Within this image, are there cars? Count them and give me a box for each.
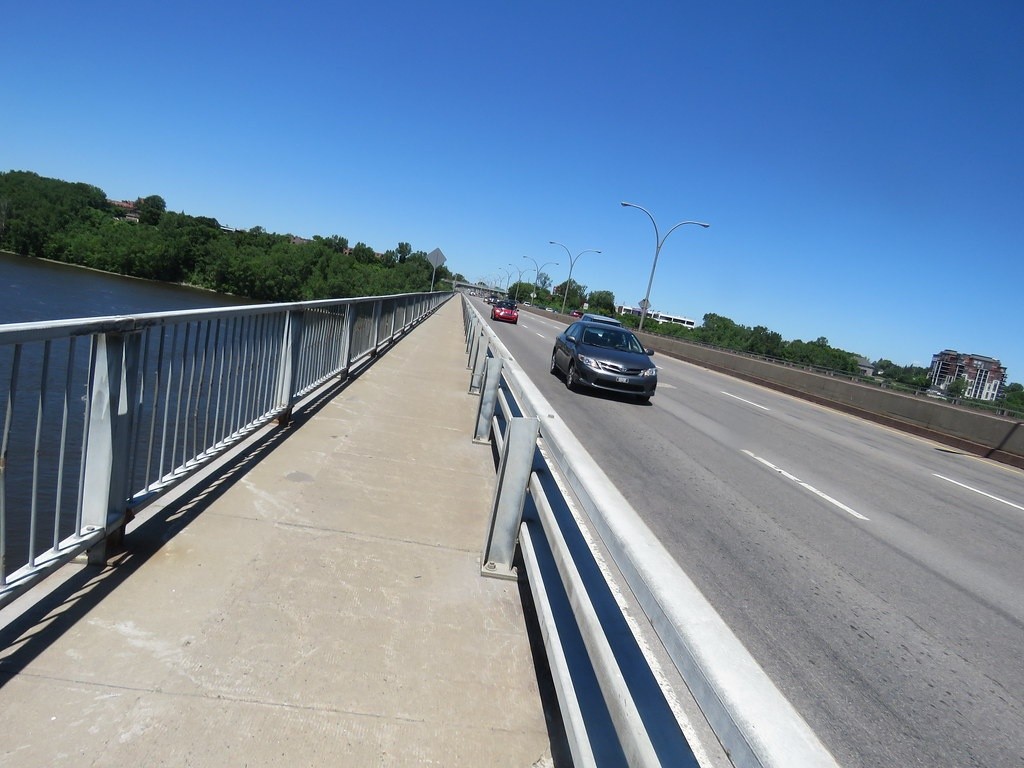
[467,290,518,309]
[491,301,520,324]
[546,307,560,314]
[523,301,531,307]
[569,310,583,318]
[549,320,658,403]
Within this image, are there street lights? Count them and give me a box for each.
[549,241,602,314]
[620,201,710,332]
[473,267,522,301]
[522,255,560,306]
[509,263,537,301]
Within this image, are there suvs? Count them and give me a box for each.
[568,313,626,344]
